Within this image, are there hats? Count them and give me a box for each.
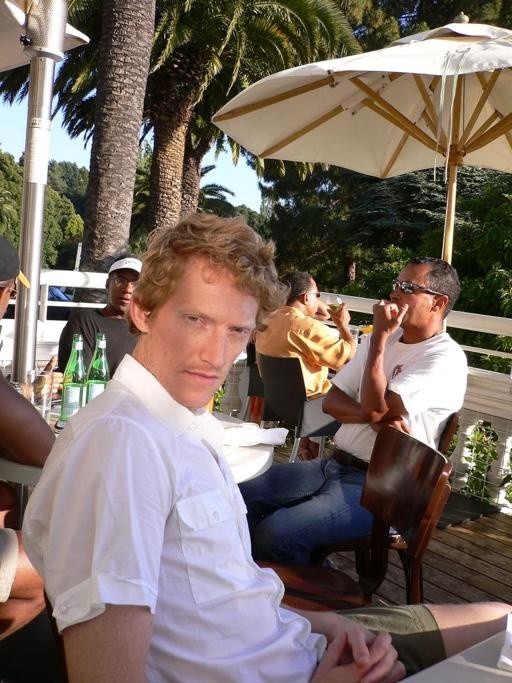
[0,236,30,288]
[109,258,142,274]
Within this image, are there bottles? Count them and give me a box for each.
[61,333,87,422]
[87,332,110,404]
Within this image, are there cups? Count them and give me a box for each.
[26,369,53,425]
[327,294,344,314]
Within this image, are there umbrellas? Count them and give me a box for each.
[210,11,512,266]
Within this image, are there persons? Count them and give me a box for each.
[60,255,143,380]
[21,210,512,682]
[1,235,55,645]
[234,257,469,611]
[255,268,357,401]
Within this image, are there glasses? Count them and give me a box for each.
[391,279,444,296]
[110,277,138,287]
[2,287,18,299]
[297,292,320,298]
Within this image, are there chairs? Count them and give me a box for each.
[256,424,453,611]
[317,411,460,596]
[241,345,265,420]
[256,353,340,461]
